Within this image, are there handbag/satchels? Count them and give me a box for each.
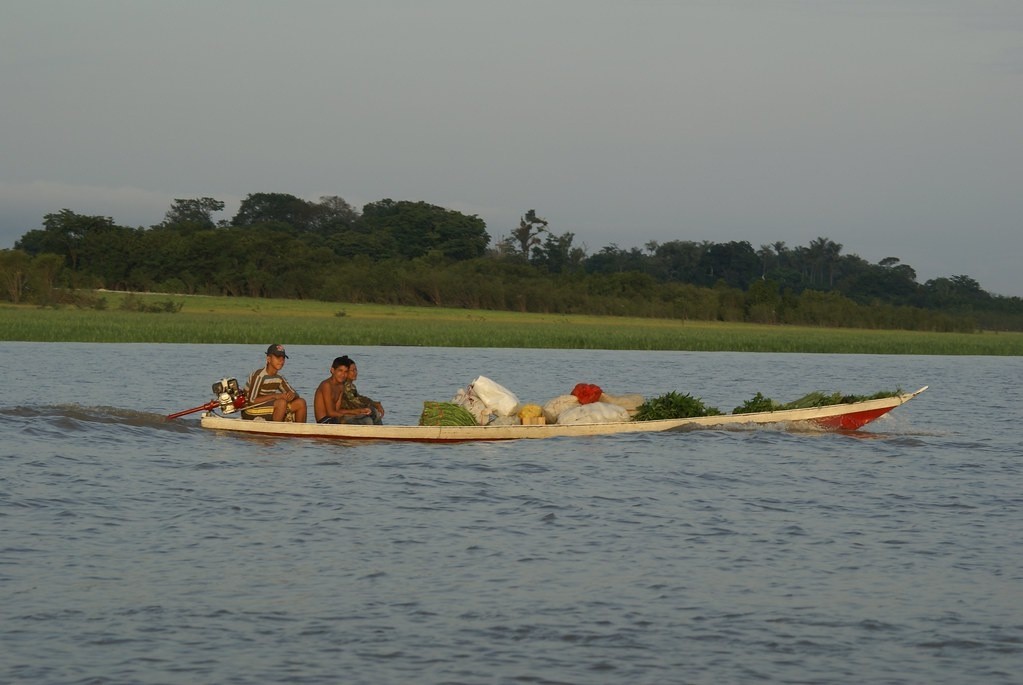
[472,375,520,417]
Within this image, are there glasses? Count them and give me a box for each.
[349,368,357,373]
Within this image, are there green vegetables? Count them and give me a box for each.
[421,400,476,426]
[631,391,727,421]
[732,386,903,414]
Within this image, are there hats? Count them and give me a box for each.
[265,344,289,359]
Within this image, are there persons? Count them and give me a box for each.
[241,344,307,422]
[343,358,385,425]
[314,357,374,424]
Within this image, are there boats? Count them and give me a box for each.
[162,377,933,444]
